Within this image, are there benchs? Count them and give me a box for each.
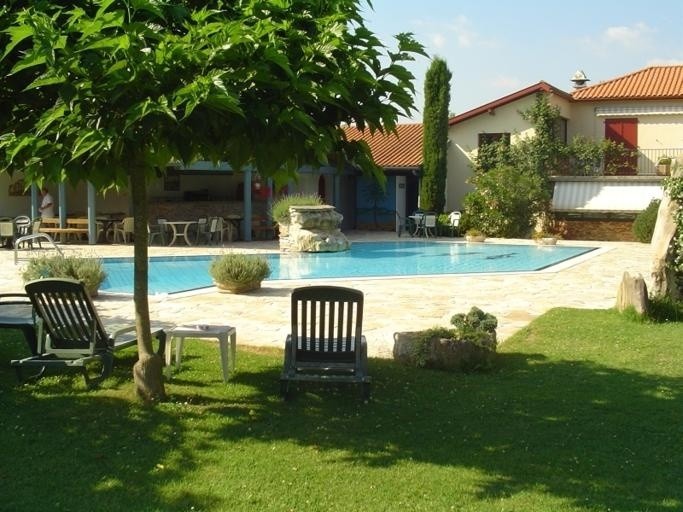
[253,217,276,239]
[38,217,90,245]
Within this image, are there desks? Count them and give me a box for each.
[172,322,237,382]
[163,220,197,246]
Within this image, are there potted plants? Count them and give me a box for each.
[210,250,271,294]
[541,232,560,245]
[656,153,673,176]
[465,228,486,242]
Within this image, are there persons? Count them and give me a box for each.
[38,186,55,237]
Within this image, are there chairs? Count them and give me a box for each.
[279,285,372,404]
[198,214,242,244]
[395,210,462,241]
[1,216,30,249]
[10,277,166,390]
[148,215,170,246]
[94,215,135,243]
